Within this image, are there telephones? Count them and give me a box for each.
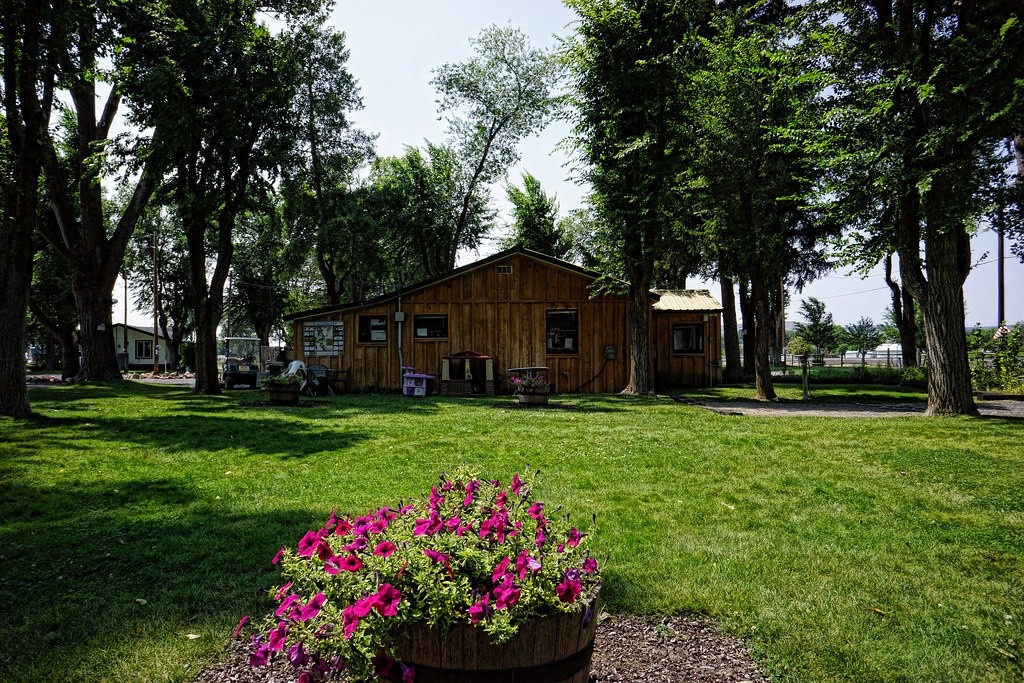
[605,345,616,360]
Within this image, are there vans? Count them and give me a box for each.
[28,347,60,367]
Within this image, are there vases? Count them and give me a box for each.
[518,387,550,407]
[390,576,599,683]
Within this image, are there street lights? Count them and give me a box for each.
[135,231,158,374]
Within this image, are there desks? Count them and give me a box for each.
[303,368,351,394]
[507,366,550,395]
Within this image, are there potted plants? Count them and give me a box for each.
[261,375,303,406]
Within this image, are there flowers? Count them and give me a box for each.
[232,472,610,683]
[507,371,551,389]
